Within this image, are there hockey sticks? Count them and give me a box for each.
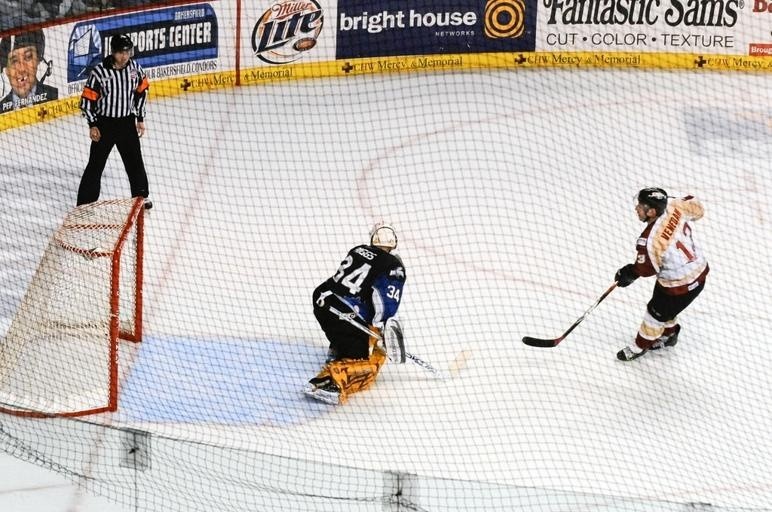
[523,195,694,347]
[331,307,474,383]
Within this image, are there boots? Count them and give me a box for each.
[617,324,681,362]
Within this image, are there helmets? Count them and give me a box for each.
[0,28,45,73]
[111,33,134,52]
[637,186,668,217]
[371,226,397,249]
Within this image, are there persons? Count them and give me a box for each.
[0,0,156,27]
[74,34,153,210]
[0,29,58,114]
[303,226,406,404]
[614,187,711,362]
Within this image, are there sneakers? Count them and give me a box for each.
[303,376,343,404]
[615,263,640,288]
[143,198,153,210]
